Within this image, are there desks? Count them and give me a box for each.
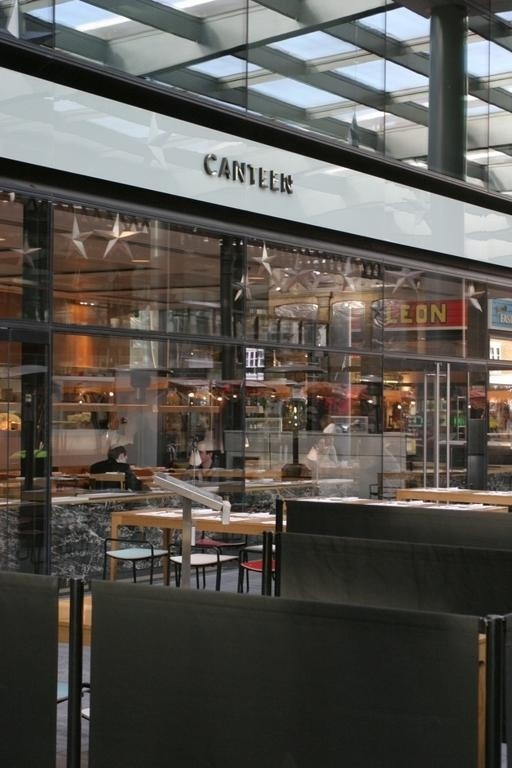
[110,507,287,586]
[288,486,512,514]
[377,467,512,500]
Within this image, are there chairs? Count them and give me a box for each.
[56,474,126,491]
[369,484,396,501]
[103,531,277,594]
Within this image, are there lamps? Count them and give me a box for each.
[306,438,319,483]
[189,438,201,486]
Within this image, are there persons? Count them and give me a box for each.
[181,440,227,482]
[308,437,342,466]
[90,444,142,491]
